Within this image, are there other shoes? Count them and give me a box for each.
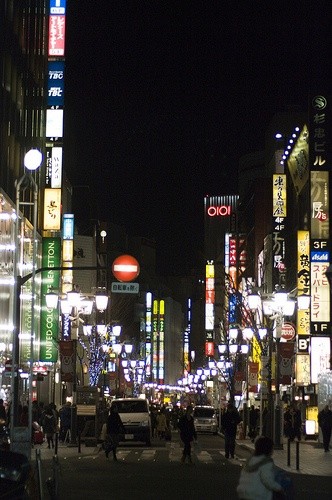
[187,458,192,464]
[181,455,185,462]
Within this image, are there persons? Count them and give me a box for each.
[149,402,186,442]
[317,405,332,453]
[215,405,301,443]
[0,397,77,449]
[220,398,242,458]
[104,404,127,461]
[178,405,198,465]
[237,434,283,500]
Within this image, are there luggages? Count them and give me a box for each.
[34,427,43,444]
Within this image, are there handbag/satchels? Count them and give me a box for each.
[193,440,201,454]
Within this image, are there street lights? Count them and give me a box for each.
[10,149,43,468]
[44,282,110,448]
[178,287,311,449]
[102,325,147,398]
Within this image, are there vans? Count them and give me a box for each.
[191,406,218,436]
[109,398,152,446]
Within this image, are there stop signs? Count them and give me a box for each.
[112,255,140,283]
